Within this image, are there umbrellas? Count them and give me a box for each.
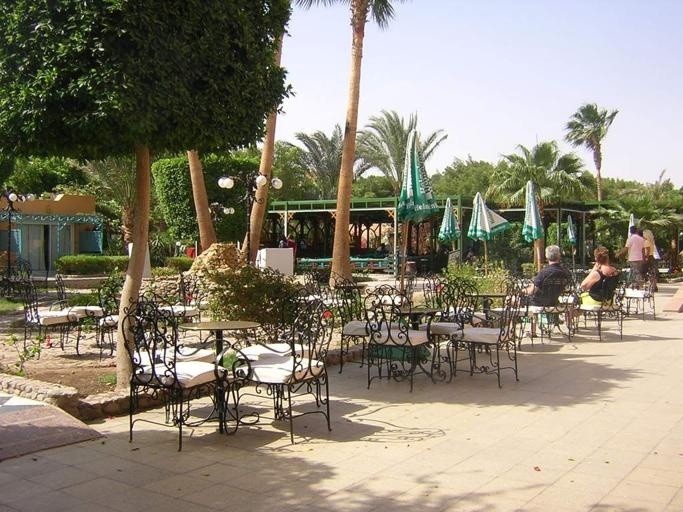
[467,191,513,276]
[566,214,577,265]
[521,179,545,274]
[396,129,440,306]
[437,196,463,251]
[626,212,636,242]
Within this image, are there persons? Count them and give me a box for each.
[613,226,644,291]
[277,234,286,248]
[286,234,297,272]
[565,245,617,329]
[349,228,367,249]
[504,244,572,338]
[636,229,660,293]
